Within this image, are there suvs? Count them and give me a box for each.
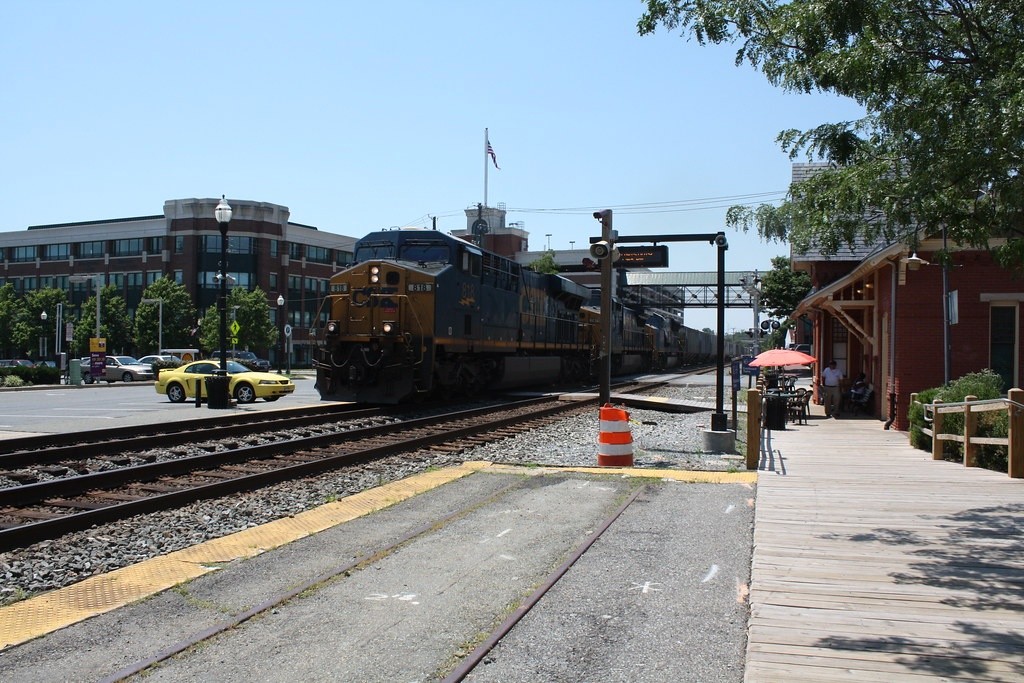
[211,351,270,372]
[0,360,34,368]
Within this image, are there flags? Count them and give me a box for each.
[487,140,499,169]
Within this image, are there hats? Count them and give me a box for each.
[827,361,837,365]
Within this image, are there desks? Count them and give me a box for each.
[762,394,803,424]
[766,390,797,419]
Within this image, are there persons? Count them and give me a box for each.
[820,360,844,418]
[841,372,865,411]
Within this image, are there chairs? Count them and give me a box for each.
[765,375,814,425]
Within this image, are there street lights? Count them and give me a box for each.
[69,274,106,384]
[215,194,233,376]
[276,295,285,374]
[143,299,162,354]
[900,215,950,390]
[39,311,47,360]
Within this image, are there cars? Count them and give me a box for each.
[80,355,150,382]
[138,355,183,369]
[156,359,293,402]
[35,360,57,368]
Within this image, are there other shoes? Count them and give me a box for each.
[836,416,840,419]
[825,415,830,419]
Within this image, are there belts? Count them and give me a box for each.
[824,385,837,387]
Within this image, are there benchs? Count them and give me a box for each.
[850,388,874,417]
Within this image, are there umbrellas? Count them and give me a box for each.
[749,347,817,391]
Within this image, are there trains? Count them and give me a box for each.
[314,230,751,408]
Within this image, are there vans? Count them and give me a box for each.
[158,348,204,365]
